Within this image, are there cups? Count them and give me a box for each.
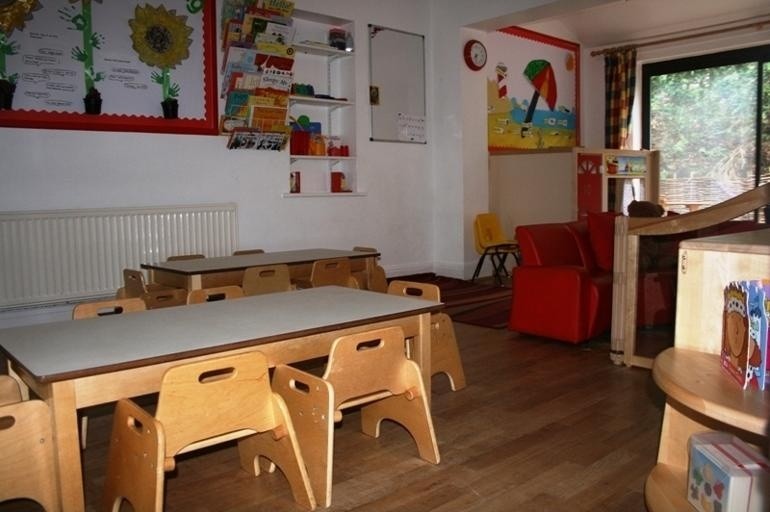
[290,172,300,193]
[331,171,345,192]
[341,145,349,157]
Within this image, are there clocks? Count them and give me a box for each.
[464,39,488,71]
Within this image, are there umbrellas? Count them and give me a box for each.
[521,60,556,138]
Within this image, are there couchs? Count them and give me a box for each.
[509,218,763,347]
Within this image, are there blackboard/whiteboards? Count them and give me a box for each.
[368,24,427,145]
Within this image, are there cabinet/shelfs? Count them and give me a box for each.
[573,147,662,217]
[220,0,365,197]
[644,348,770,512]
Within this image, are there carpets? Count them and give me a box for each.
[387,273,512,329]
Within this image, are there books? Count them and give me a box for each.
[218,0,296,151]
[687,431,770,512]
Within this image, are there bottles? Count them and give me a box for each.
[345,32,354,52]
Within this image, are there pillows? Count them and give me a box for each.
[587,210,623,274]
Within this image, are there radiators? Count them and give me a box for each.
[0,203,240,311]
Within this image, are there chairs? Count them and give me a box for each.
[471,213,519,287]
